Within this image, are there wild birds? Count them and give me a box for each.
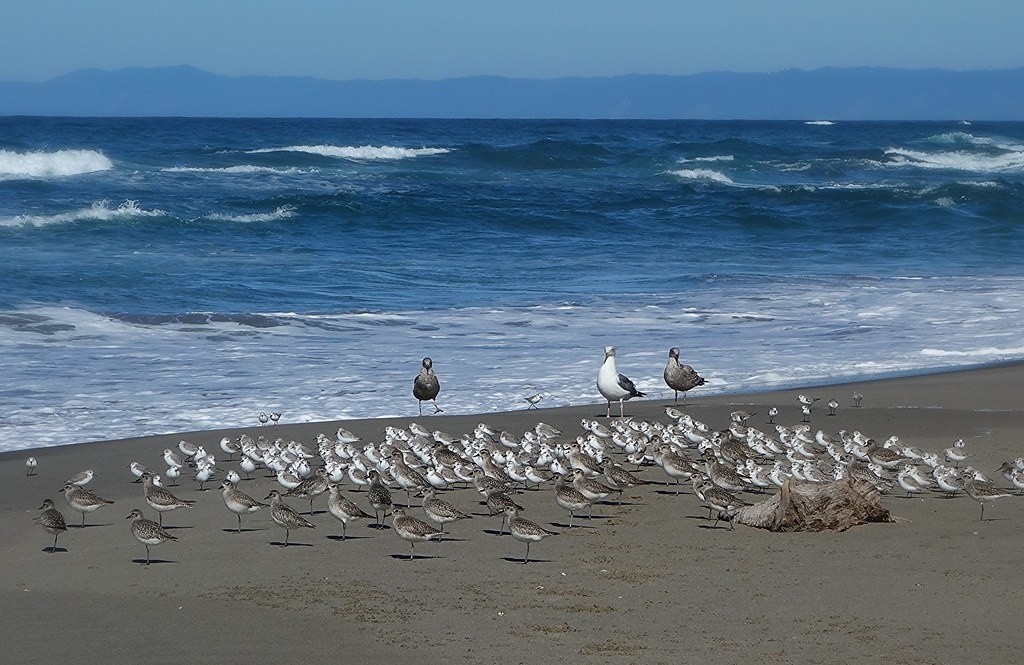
[796,389,863,421]
[324,483,377,541]
[263,490,317,547]
[490,505,560,563]
[26,456,38,475]
[259,411,281,427]
[664,347,708,408]
[652,403,1024,534]
[333,417,651,529]
[412,357,444,416]
[413,486,474,542]
[59,483,114,527]
[384,506,450,562]
[524,394,544,410]
[33,499,68,553]
[125,509,178,564]
[597,345,647,419]
[126,434,334,533]
